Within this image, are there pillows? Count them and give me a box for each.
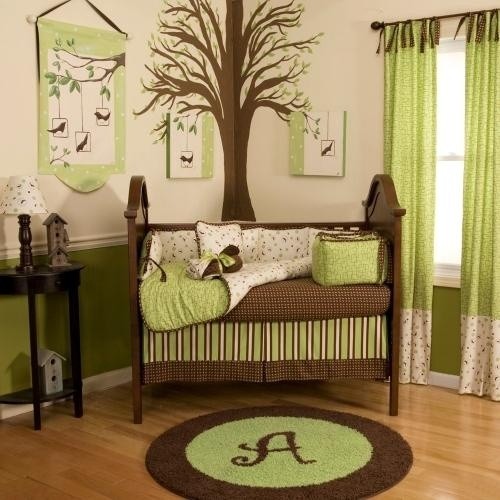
[137,228,162,281]
[195,220,245,259]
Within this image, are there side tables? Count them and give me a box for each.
[0,261,87,431]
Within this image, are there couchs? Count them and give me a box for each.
[124,174,406,425]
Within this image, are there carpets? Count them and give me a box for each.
[145,405,413,500]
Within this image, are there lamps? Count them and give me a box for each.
[0,175,48,275]
